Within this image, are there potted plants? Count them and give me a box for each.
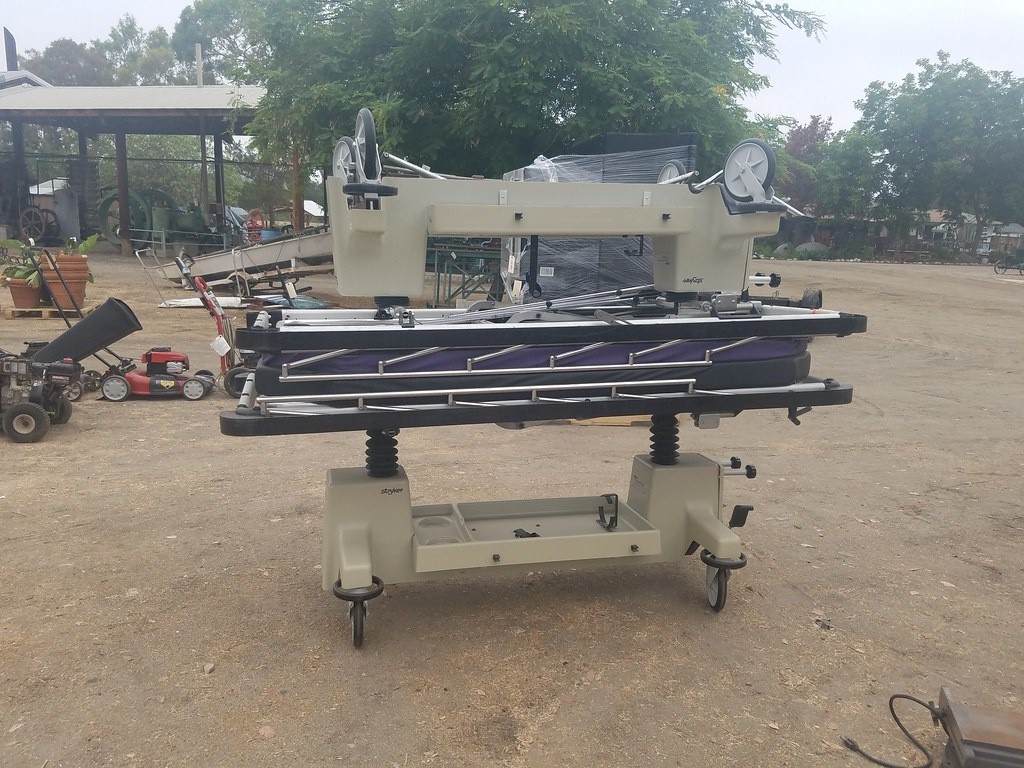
[0,231,100,308]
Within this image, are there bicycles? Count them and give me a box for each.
[994,249,1024,275]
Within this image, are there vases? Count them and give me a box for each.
[41,253,90,309]
